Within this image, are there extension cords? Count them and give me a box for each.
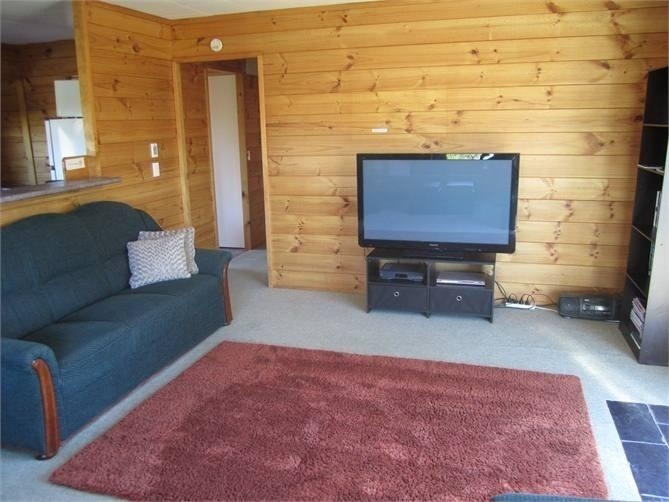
[505,299,535,309]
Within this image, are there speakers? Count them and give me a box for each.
[558,295,618,321]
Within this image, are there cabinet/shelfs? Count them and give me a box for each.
[366,252,493,322]
[618,66,669,366]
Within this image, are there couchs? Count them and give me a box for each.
[1,201,232,460]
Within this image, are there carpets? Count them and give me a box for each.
[609,401,668,501]
[51,339,609,502]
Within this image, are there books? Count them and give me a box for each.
[637,163,664,171]
[629,295,646,352]
[647,242,654,298]
[651,189,663,242]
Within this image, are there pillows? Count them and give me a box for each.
[138,226,198,275]
[127,234,191,289]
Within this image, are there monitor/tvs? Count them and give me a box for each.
[357,153,520,259]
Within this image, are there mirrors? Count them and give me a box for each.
[1,0,89,196]
